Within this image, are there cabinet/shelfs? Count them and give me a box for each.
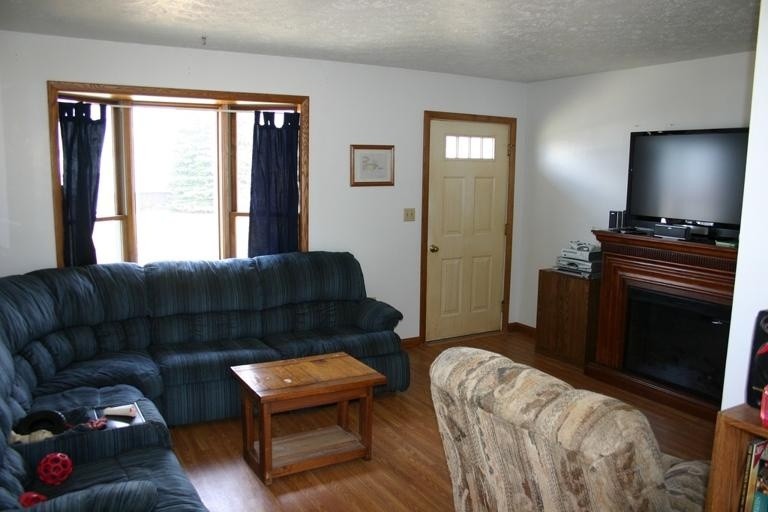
[535,265,597,366]
[587,228,738,421]
[704,401,768,511]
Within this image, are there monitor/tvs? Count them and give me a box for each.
[625,127,750,245]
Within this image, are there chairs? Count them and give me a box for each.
[427,345,713,511]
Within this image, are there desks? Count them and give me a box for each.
[224,347,389,484]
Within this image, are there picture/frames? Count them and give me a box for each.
[349,143,396,189]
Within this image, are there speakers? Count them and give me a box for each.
[608,211,622,231]
[623,210,635,231]
[653,224,691,241]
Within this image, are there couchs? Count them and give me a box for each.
[3,249,413,511]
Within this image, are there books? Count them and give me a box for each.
[737,440,768,512]
